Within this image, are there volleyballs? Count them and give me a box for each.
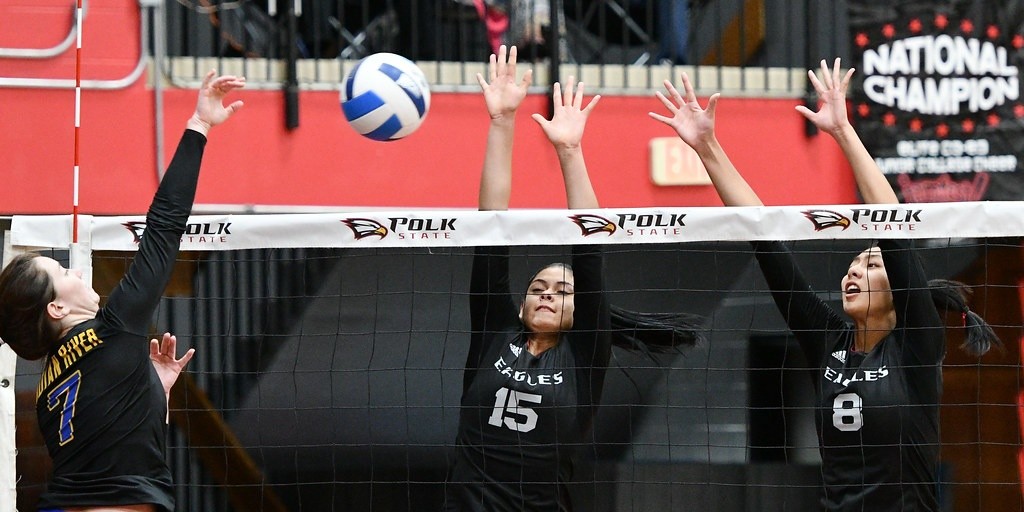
[339,53,431,143]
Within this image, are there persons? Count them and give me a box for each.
[0,69,245,512]
[648,58,1003,512]
[387,0,696,67]
[444,44,706,511]
